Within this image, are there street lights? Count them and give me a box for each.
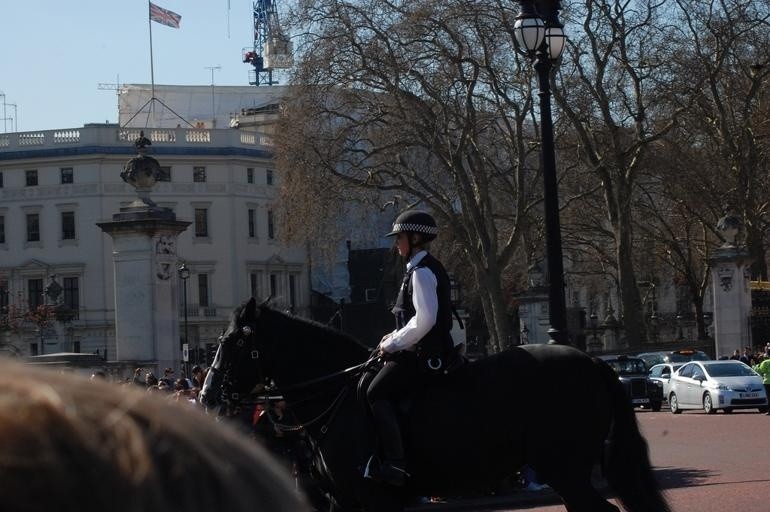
[522,321,529,345]
[590,312,599,343]
[514,0,568,346]
[180,261,191,345]
[642,280,713,342]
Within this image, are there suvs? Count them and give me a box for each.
[599,353,663,412]
[636,347,712,372]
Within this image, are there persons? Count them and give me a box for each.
[85,364,205,401]
[356,210,455,480]
[720,343,770,413]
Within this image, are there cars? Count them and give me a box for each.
[648,362,689,401]
[666,360,769,415]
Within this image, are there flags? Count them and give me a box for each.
[151,3,182,28]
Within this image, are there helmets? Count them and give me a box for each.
[385,210,439,239]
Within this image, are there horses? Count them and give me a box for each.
[197,293,676,512]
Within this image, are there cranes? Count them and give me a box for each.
[242,0,298,87]
